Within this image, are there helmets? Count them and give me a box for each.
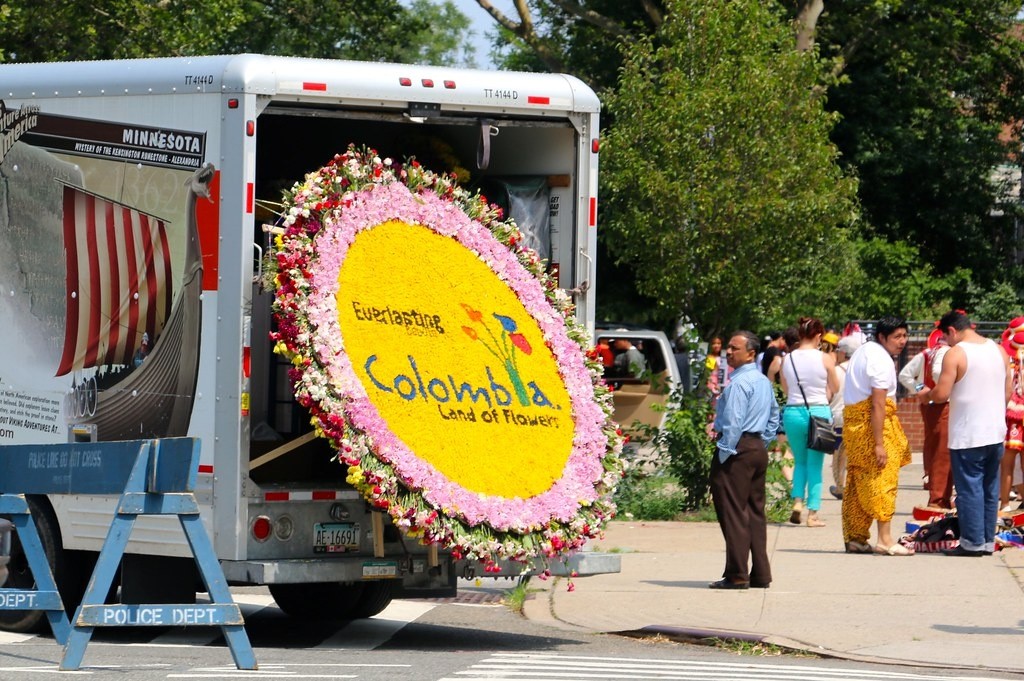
[822,332,839,346]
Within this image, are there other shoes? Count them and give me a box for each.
[943,546,982,557]
[807,518,826,527]
[830,485,843,499]
[708,578,750,589]
[789,503,802,525]
[983,550,993,556]
[750,582,769,588]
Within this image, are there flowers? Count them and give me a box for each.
[258,144,639,600]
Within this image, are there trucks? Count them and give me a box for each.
[0,51,602,645]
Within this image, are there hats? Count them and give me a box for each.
[1004,314,1024,337]
[1003,331,1024,359]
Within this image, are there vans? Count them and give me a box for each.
[592,326,686,476]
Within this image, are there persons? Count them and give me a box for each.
[702,317,915,589]
[1002,316,1024,526]
[898,309,1012,558]
[443,153,510,221]
[598,326,688,380]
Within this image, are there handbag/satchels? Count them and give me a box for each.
[806,414,836,455]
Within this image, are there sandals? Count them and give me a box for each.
[846,541,875,554]
[875,542,915,556]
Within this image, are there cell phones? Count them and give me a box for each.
[916,384,933,404]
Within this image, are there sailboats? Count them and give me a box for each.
[52,160,215,442]
